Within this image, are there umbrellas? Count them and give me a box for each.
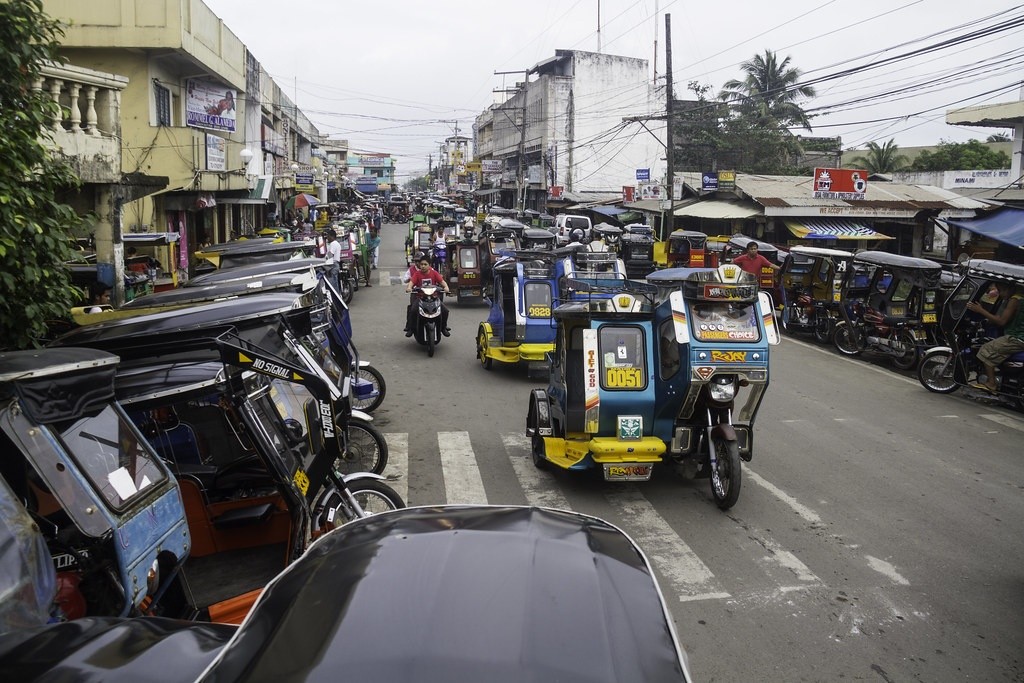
[285,193,321,209]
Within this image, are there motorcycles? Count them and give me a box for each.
[404,283,460,355]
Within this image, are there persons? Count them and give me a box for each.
[303,218,313,232]
[586,231,608,252]
[199,235,211,250]
[325,229,341,269]
[228,230,237,243]
[298,211,303,221]
[126,247,136,259]
[966,281,1024,389]
[731,242,780,284]
[309,207,327,221]
[392,205,398,224]
[403,252,451,337]
[464,212,477,235]
[89,282,111,313]
[566,234,582,247]
[431,225,448,249]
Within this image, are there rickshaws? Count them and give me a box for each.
[915,259,1024,410]
[779,244,856,346]
[520,263,793,508]
[831,249,945,373]
[48,182,786,574]
[0,348,210,659]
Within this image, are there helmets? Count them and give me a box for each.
[411,252,425,261]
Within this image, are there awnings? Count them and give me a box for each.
[783,217,896,239]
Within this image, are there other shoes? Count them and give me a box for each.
[404,327,409,331]
[441,329,450,337]
[445,326,451,331]
[405,329,413,337]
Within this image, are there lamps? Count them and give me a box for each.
[239,149,254,170]
[290,163,299,174]
